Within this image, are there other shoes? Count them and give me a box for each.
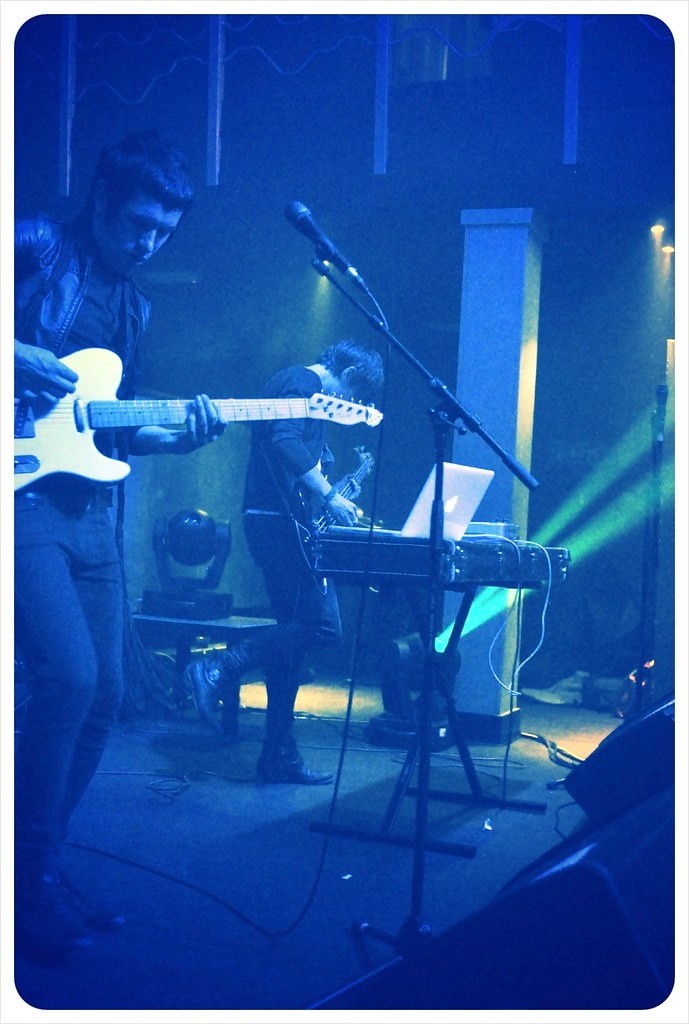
[13,876,79,962]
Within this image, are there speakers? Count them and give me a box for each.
[302,784,674,1009]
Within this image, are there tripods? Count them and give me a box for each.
[308,331,547,944]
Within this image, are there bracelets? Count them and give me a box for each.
[320,487,337,510]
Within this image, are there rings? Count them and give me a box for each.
[217,418,228,430]
[352,518,358,523]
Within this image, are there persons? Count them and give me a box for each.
[13,130,226,951]
[182,338,387,786]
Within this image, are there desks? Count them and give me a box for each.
[131,614,279,741]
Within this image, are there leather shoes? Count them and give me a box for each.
[182,653,224,735]
[257,752,333,784]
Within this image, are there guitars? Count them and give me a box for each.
[14,347,385,493]
[288,446,376,602]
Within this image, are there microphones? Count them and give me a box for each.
[310,257,371,326]
[285,199,369,296]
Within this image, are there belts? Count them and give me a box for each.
[24,482,114,517]
